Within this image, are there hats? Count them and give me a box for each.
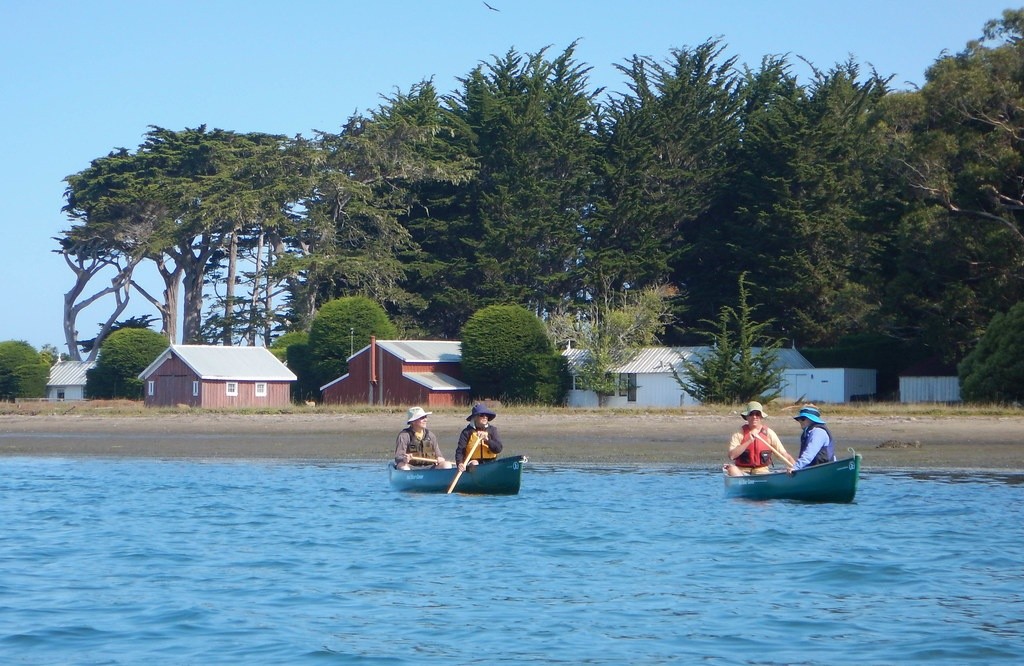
[741,401,769,421]
[466,405,496,421]
[793,407,826,426]
[407,407,433,425]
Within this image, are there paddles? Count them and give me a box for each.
[446,435,484,495]
[751,432,794,468]
[405,453,457,465]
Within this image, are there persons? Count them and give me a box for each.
[727,401,795,476]
[787,406,836,476]
[395,407,452,470]
[455,404,502,472]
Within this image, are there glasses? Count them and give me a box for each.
[749,413,760,416]
[796,418,806,422]
[478,414,488,417]
